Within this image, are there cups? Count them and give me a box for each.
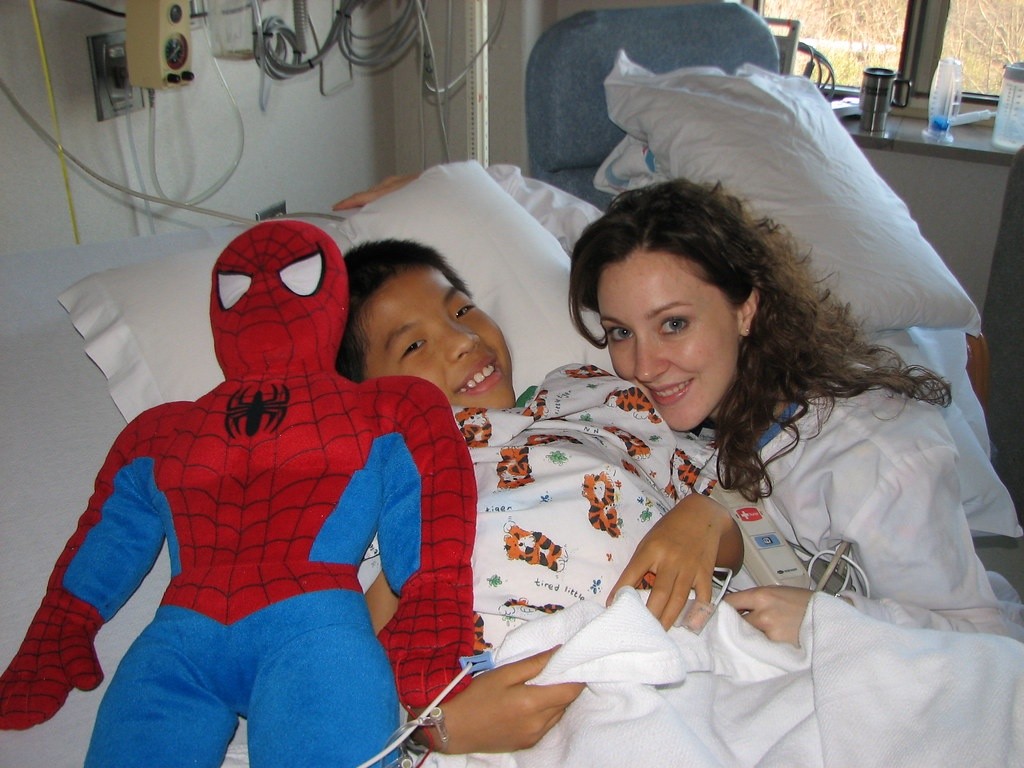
[860,67,913,131]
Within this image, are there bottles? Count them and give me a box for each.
[992,62,1024,154]
[922,57,964,144]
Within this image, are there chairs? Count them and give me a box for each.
[525,2,782,210]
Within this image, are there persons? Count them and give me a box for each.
[335,237,747,755]
[568,180,1024,652]
[1,219,478,768]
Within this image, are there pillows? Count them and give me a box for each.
[52,156,607,452]
[605,49,981,341]
